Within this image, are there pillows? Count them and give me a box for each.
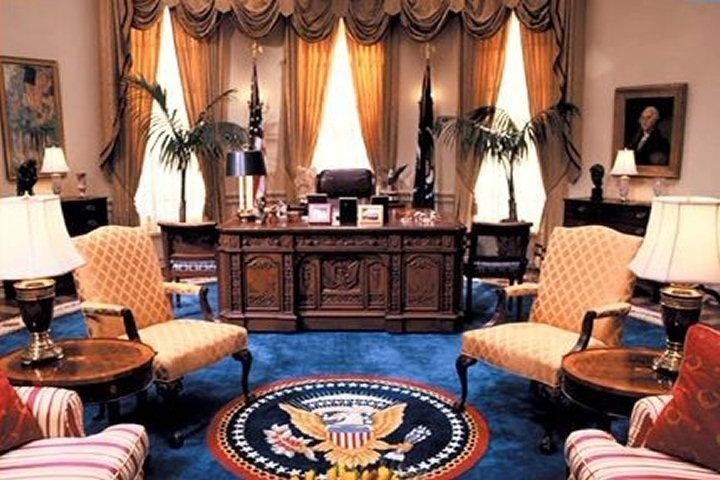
[0,378,44,452]
[644,325,719,473]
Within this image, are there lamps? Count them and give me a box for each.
[39,146,72,194]
[225,150,267,222]
[611,148,639,203]
[0,191,88,368]
[625,196,720,385]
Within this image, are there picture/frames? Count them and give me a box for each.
[306,194,327,204]
[1,55,68,182]
[356,204,384,226]
[609,82,688,179]
[371,196,389,223]
[338,197,358,223]
[307,203,333,225]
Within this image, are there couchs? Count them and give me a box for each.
[1,386,152,480]
[564,321,720,479]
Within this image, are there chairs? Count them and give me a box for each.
[462,223,533,321]
[451,225,646,454]
[315,168,373,209]
[157,222,218,309]
[71,224,255,449]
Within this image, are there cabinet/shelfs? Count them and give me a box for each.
[62,196,108,237]
[561,196,652,238]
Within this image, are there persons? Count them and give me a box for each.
[630,107,669,166]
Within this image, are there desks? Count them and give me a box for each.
[215,209,467,334]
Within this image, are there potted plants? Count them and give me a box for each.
[432,102,583,262]
